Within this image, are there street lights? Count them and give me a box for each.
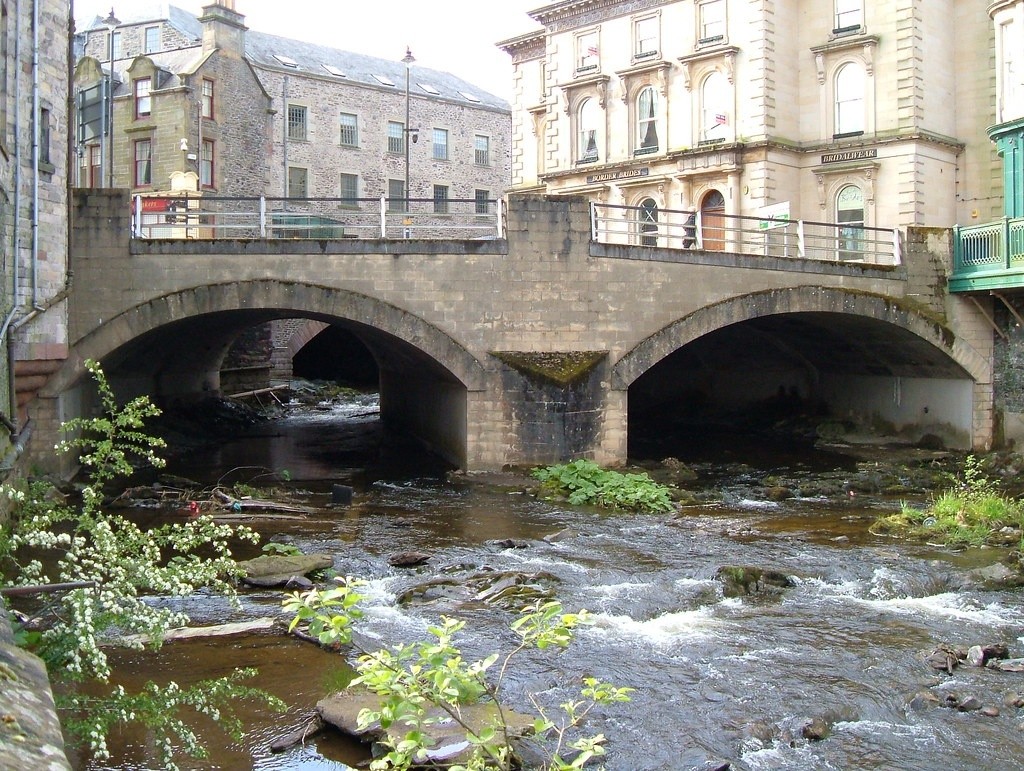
[101,7,121,190]
[400,46,417,238]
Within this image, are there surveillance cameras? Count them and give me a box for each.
[413,134,418,144]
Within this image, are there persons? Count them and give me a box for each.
[682,207,695,248]
